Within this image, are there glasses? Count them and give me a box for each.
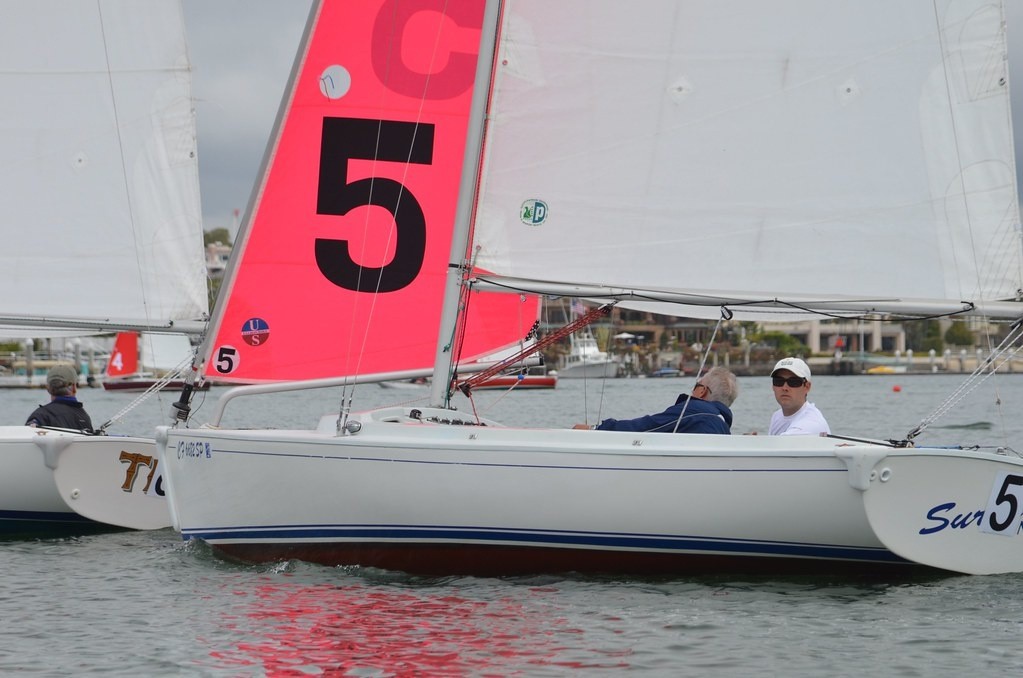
[773,376,807,388]
[693,382,712,394]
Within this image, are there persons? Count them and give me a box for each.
[752,357,832,435]
[25,364,95,433]
[571,364,738,435]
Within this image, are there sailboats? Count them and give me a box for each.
[1,0,208,534]
[96,326,212,394]
[453,338,558,392]
[155,0,1023,585]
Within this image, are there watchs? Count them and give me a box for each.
[590,424,597,430]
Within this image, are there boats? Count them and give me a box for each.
[647,361,683,378]
[552,297,621,379]
[378,381,431,391]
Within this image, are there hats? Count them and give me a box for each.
[770,357,811,382]
[47,364,81,383]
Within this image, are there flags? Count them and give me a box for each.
[570,298,584,315]
